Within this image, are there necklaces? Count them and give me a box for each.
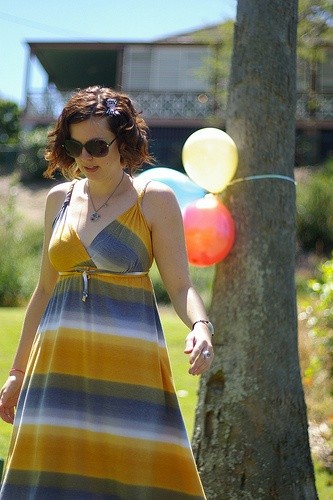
[86,169,125,222]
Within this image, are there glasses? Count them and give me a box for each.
[60,134,118,157]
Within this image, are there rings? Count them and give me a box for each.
[201,350,211,359]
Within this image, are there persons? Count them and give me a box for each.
[0,83,216,499]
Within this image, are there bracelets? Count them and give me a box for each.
[192,320,215,337]
[8,368,25,376]
